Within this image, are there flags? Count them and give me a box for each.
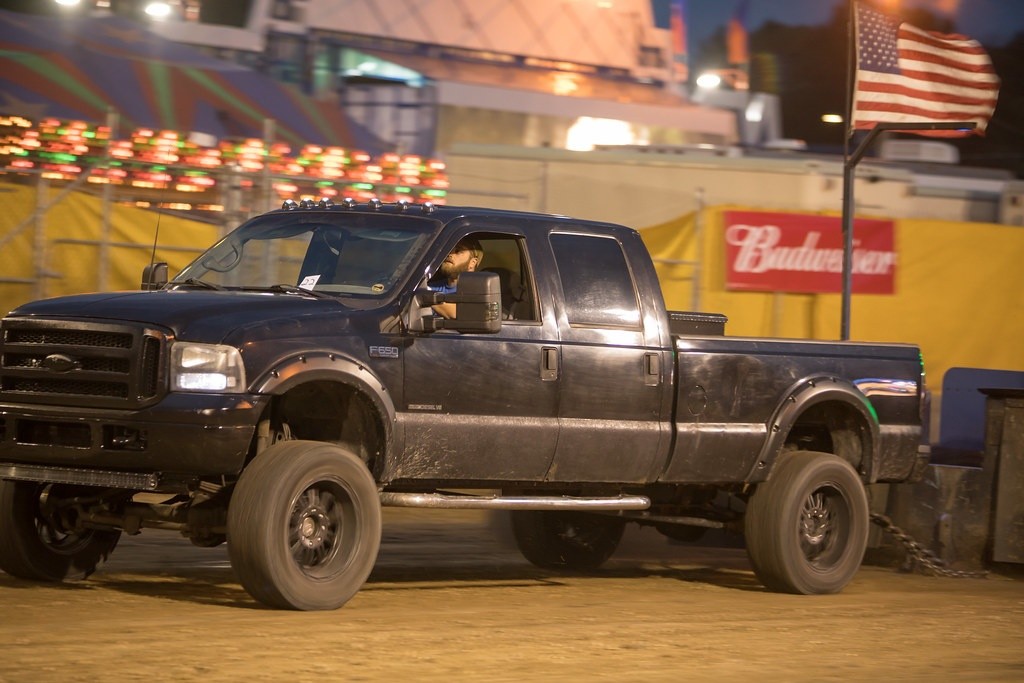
[851,2,1001,139]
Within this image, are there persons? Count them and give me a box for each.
[431,235,514,320]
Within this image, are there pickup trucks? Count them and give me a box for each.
[0,199,931,606]
